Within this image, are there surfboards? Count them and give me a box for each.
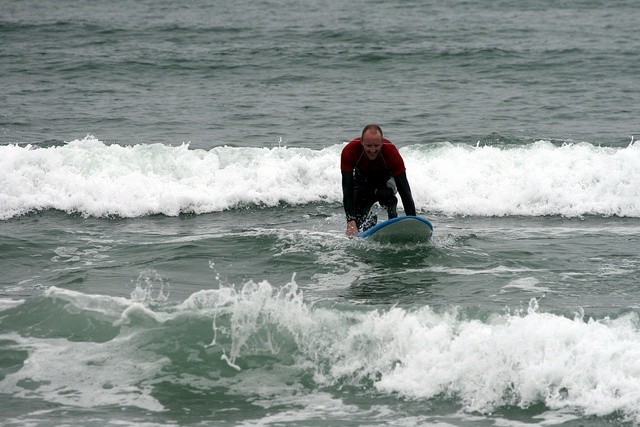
[357,216,432,242]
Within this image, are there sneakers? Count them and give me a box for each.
[363,215,377,231]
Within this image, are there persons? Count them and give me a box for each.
[341,124,416,239]
